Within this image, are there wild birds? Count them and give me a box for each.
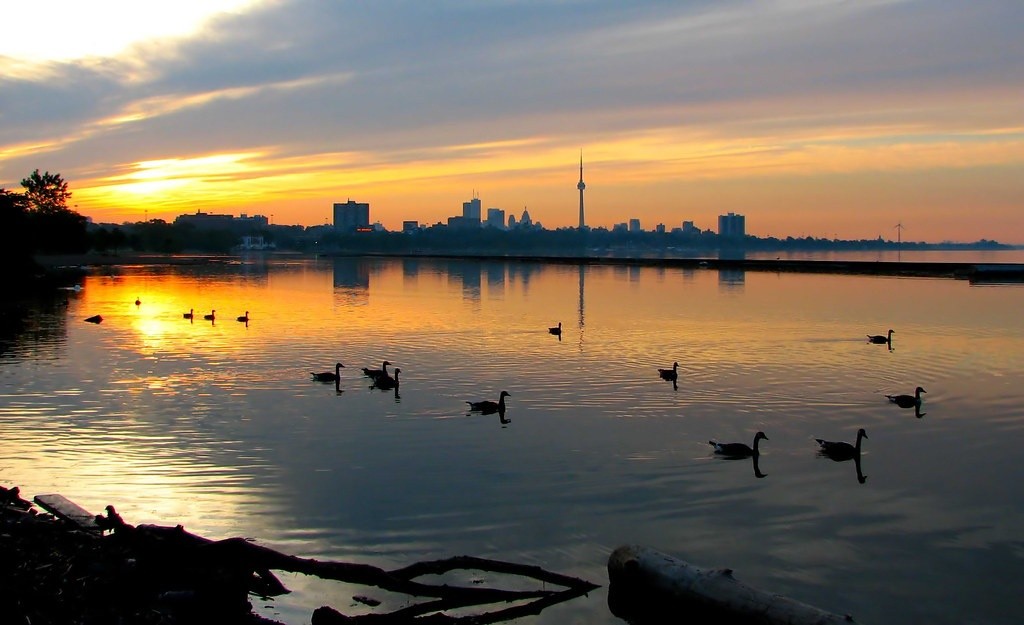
[707,431,770,455]
[465,390,512,408]
[237,310,250,321]
[204,309,216,319]
[815,428,869,453]
[311,362,345,378]
[361,360,402,382]
[866,329,896,345]
[183,309,193,318]
[135,296,141,306]
[548,322,563,333]
[885,386,927,405]
[657,361,680,375]
